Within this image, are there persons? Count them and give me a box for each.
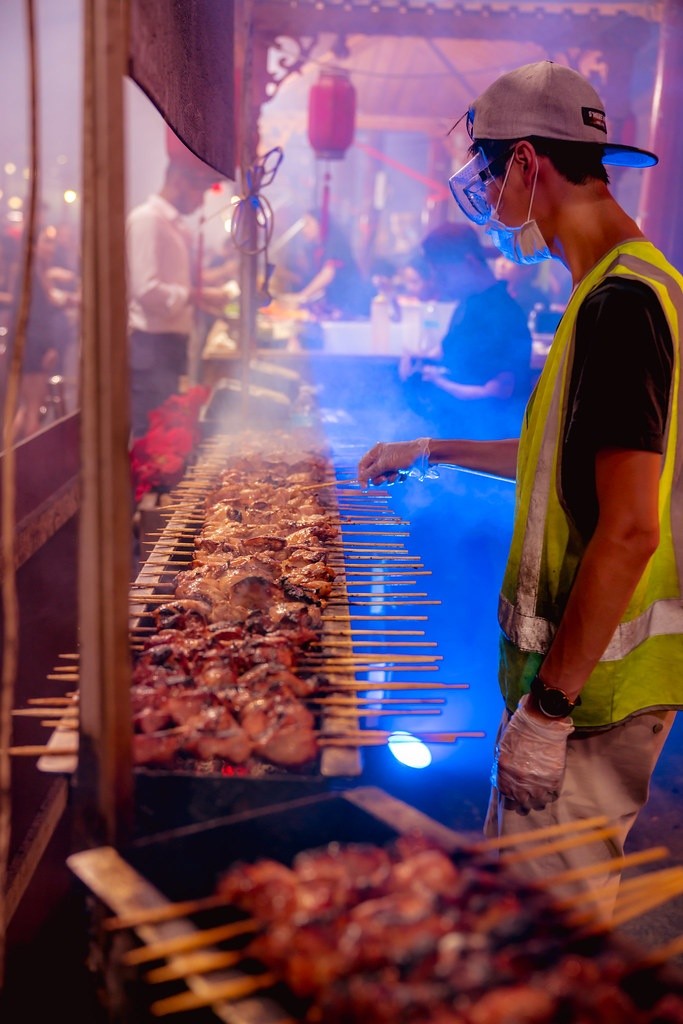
[399,219,533,495]
[274,208,572,326]
[350,64,682,927]
[126,160,241,428]
[0,202,80,432]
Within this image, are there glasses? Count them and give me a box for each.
[460,144,516,218]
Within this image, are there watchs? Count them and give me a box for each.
[527,672,583,719]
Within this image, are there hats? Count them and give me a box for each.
[467,61,659,168]
[422,221,504,266]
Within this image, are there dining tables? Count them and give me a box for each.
[201,320,552,371]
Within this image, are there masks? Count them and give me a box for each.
[483,151,554,264]
